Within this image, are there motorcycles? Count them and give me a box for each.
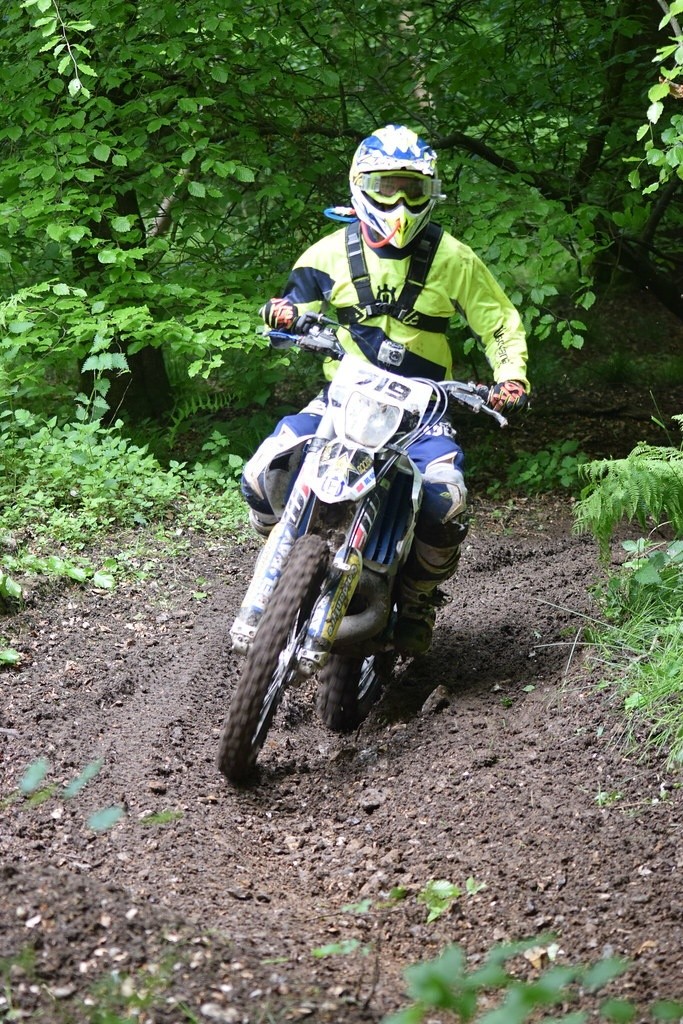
[212,312,529,788]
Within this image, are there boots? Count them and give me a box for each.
[393,547,460,655]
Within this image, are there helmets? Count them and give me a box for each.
[349,124,442,248]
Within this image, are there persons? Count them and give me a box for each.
[237,124,528,658]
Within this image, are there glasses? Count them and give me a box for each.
[365,172,432,206]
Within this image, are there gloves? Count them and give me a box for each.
[264,298,297,328]
[489,380,529,418]
